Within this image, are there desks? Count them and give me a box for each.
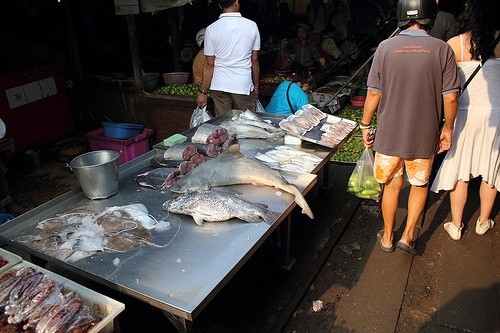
[0,108,359,333]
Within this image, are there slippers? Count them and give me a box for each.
[396,241,418,256]
[377,230,394,252]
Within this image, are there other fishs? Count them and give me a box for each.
[170,143,315,219]
[163,189,281,226]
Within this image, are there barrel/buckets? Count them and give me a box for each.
[0,213,16,246]
[66,149,120,200]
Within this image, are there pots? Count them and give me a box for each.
[51,136,87,162]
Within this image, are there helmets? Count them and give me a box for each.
[397,0,439,35]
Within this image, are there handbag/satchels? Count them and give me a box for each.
[256,100,266,113]
[190,106,213,129]
[346,146,382,202]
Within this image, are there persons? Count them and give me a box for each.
[196,0,260,117]
[360,0,461,255]
[319,24,342,74]
[192,28,206,83]
[264,62,309,114]
[430,0,500,240]
[428,0,479,41]
[279,23,326,69]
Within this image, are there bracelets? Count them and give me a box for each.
[360,121,372,128]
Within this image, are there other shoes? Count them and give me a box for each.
[475,217,494,235]
[444,221,464,240]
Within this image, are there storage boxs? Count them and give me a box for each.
[84,126,154,165]
[313,86,350,104]
[0,247,125,333]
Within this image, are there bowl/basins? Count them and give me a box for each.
[350,96,366,107]
[161,72,190,85]
[101,120,144,139]
[132,72,160,92]
